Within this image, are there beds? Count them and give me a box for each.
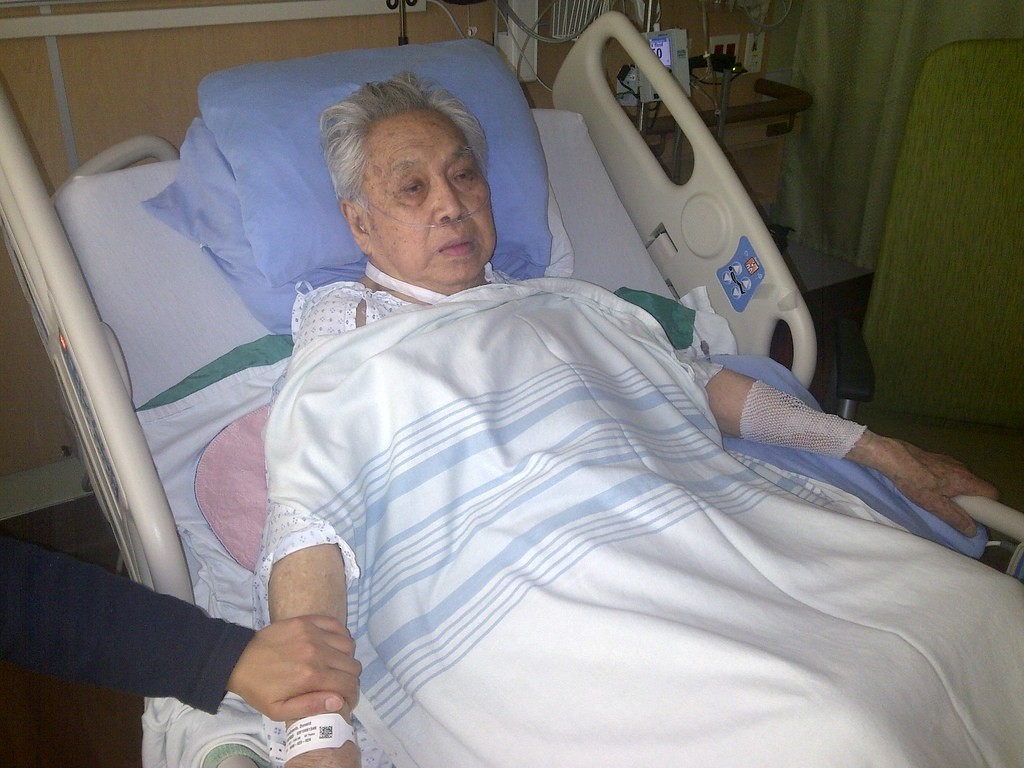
[0,0,1024,768]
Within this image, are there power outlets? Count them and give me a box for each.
[743,32,764,72]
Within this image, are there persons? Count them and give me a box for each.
[0,537,362,722]
[264,70,1024,768]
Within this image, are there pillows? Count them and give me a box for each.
[192,37,551,281]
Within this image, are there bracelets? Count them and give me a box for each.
[284,714,357,763]
